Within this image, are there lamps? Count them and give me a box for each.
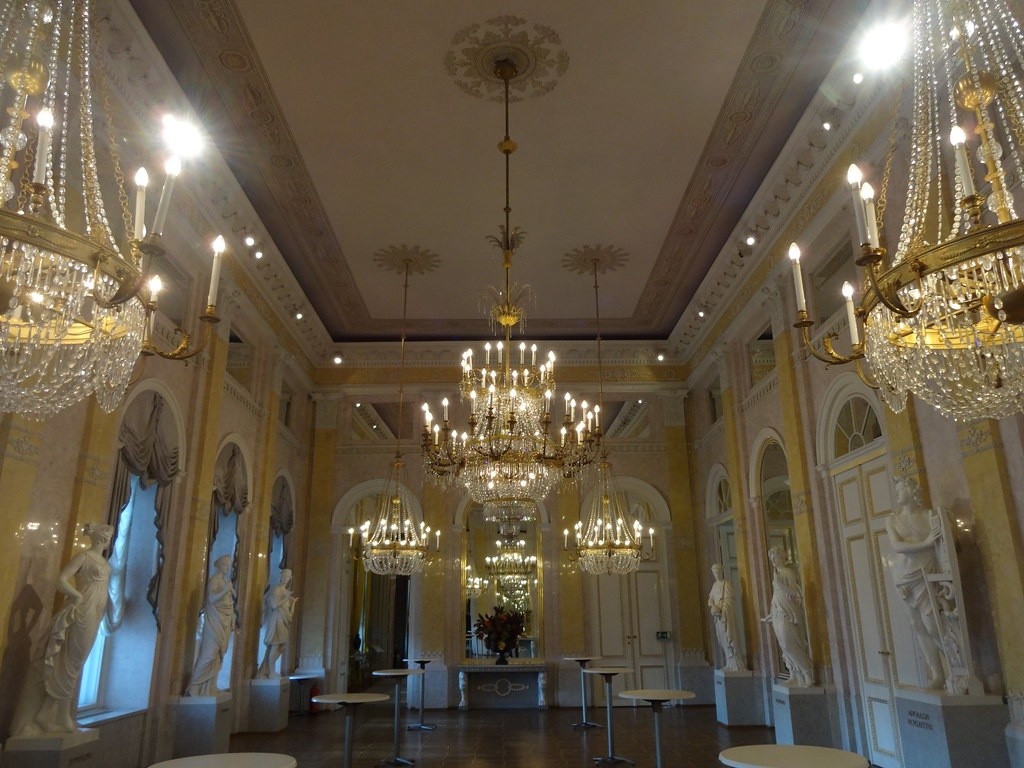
[785,0,1024,419]
[467,524,537,610]
[562,259,656,577]
[362,259,442,574]
[418,59,578,548]
[0,1,226,421]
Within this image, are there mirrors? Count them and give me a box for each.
[461,499,544,665]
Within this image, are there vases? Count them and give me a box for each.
[485,639,517,665]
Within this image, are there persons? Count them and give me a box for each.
[32,520,115,734]
[764,546,817,688]
[182,555,237,697]
[707,565,748,672]
[258,568,299,679]
[882,473,959,690]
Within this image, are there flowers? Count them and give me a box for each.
[474,605,531,654]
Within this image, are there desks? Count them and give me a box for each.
[371,669,426,768]
[619,689,696,768]
[563,658,605,729]
[458,665,550,712]
[402,659,441,730]
[148,752,297,768]
[312,693,390,768]
[583,668,636,766]
[718,745,869,768]
